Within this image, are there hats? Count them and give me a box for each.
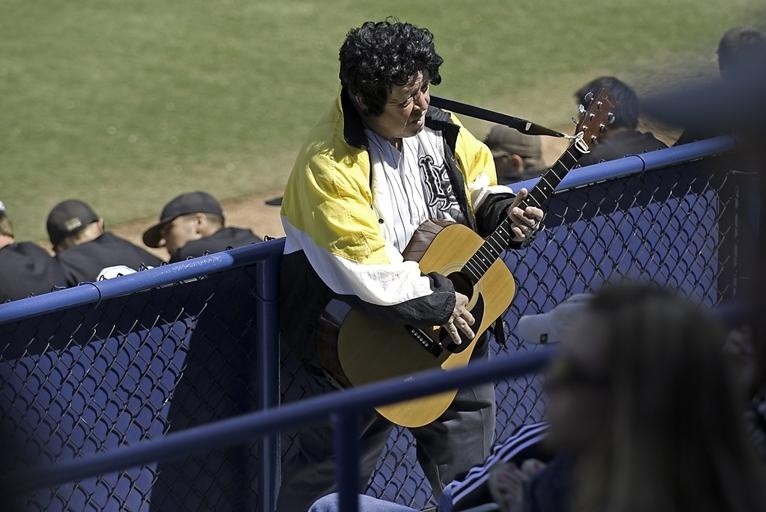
[518,294,595,343]
[142,192,224,247]
[47,200,99,248]
[483,122,541,157]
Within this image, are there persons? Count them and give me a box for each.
[46,200,166,286]
[573,76,669,166]
[0,202,69,300]
[280,18,544,511]
[673,27,766,146]
[482,124,544,184]
[142,194,264,266]
[309,278,765,510]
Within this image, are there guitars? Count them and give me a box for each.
[316,89,622,430]
